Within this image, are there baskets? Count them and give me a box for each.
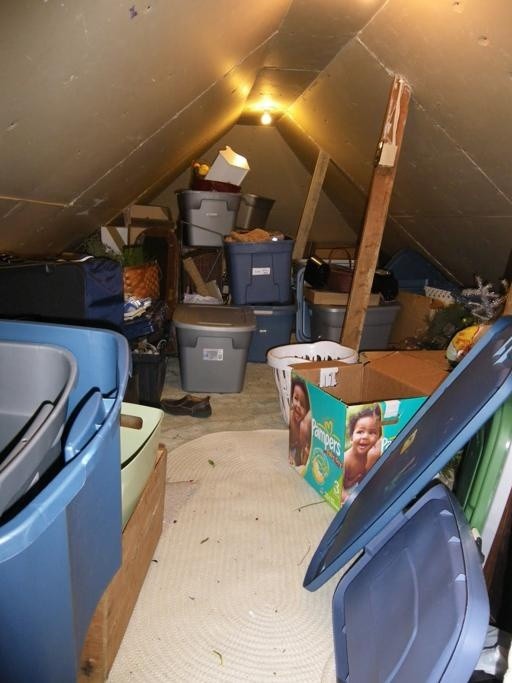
[123,259,161,310]
[267,339,358,427]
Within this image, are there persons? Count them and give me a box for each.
[342,410,381,499]
[291,381,312,465]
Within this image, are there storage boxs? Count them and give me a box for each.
[0,320,171,681]
[222,233,295,304]
[2,190,175,320]
[232,298,299,363]
[401,280,463,298]
[287,354,455,511]
[307,301,402,354]
[172,302,255,394]
[177,191,243,249]
[310,240,355,260]
[298,286,382,306]
[183,252,225,303]
[389,291,445,351]
[305,256,400,300]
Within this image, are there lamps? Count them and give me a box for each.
[261,108,272,125]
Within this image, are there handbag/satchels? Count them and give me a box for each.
[304,255,330,288]
[1,251,125,329]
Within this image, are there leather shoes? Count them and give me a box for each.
[160,394,212,417]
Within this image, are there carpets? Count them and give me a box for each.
[107,428,347,683]
